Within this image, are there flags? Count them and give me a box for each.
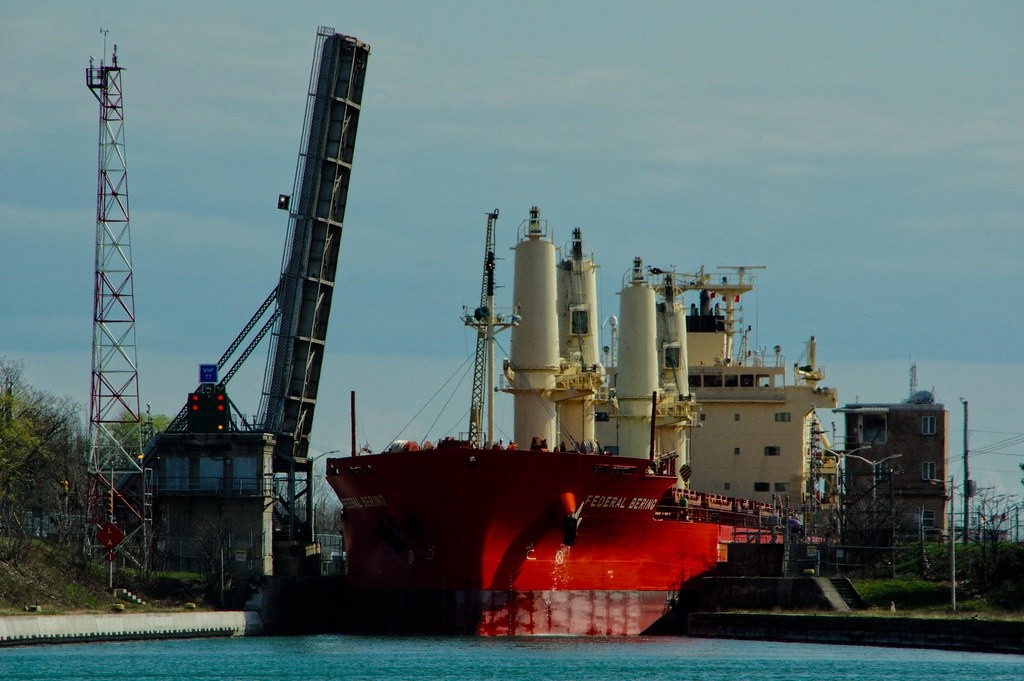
[722,294,740,302]
[708,292,715,299]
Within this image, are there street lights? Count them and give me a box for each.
[931,476,957,610]
[846,453,902,528]
[822,445,871,524]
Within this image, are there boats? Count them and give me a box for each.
[325,207,843,637]
